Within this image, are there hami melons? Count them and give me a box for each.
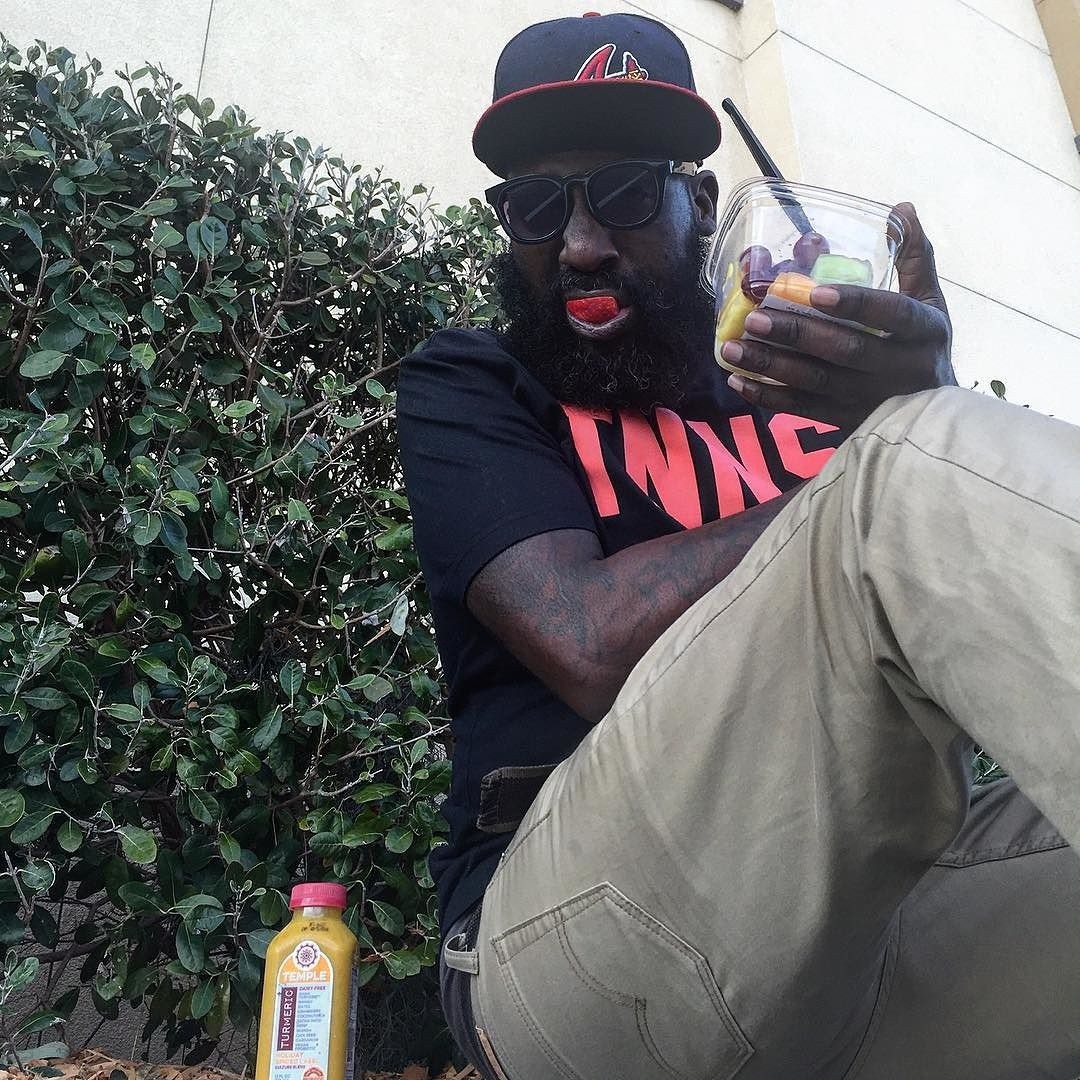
[766,271,822,308]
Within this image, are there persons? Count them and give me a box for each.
[392,9,1080,1080]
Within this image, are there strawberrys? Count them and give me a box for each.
[567,296,619,324]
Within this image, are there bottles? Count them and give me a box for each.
[253,882,357,1078]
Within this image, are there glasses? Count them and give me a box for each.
[485,158,699,245]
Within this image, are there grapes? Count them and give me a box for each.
[737,232,830,302]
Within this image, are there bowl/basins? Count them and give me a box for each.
[698,176,912,388]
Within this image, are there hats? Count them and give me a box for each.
[472,13,720,178]
